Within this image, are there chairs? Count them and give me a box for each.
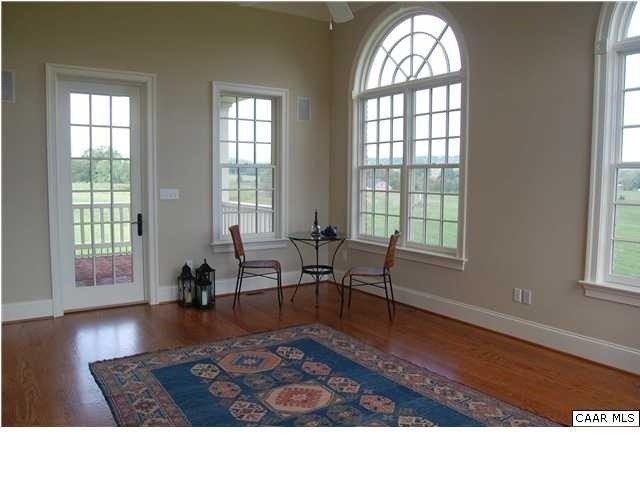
[340,230,400,322]
[229,225,282,307]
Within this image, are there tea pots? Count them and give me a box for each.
[321,225,338,236]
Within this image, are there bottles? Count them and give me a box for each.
[311,208,321,237]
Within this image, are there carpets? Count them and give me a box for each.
[89,322,569,426]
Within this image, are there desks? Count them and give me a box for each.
[289,232,345,302]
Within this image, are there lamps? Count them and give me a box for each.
[326,2,353,24]
[196,258,215,309]
[178,262,196,307]
